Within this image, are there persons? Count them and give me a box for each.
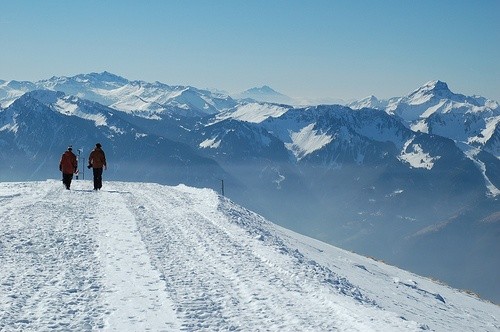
[59,146,78,190]
[88,143,107,190]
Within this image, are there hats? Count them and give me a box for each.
[68,145,72,149]
[96,142,101,147]
